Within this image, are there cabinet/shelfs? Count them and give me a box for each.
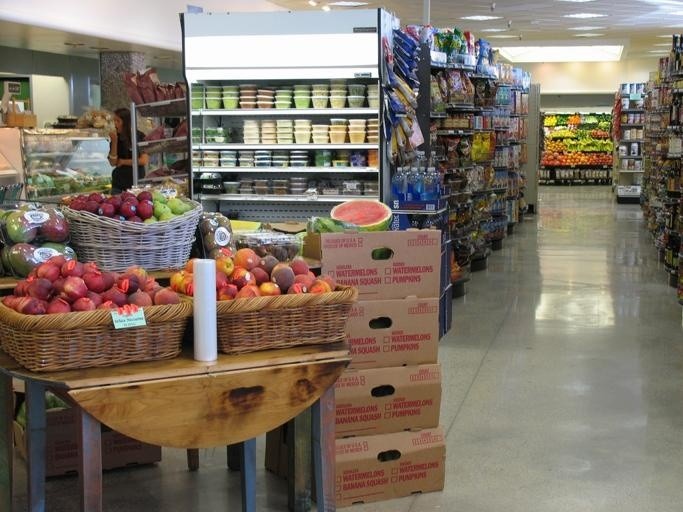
[614,31,683,305]
[539,110,615,185]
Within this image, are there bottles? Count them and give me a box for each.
[391,151,449,241]
[664,33,683,277]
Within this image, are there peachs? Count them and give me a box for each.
[3,253,181,316]
[170,247,335,300]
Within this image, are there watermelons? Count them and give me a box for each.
[293,199,393,261]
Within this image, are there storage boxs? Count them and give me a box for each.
[335,363,441,440]
[336,426,447,508]
[345,295,441,368]
[13,378,162,480]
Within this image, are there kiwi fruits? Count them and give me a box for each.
[192,212,298,260]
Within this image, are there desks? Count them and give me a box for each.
[0,339,354,511]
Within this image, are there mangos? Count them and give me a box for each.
[0,204,77,279]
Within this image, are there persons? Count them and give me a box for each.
[108,108,148,195]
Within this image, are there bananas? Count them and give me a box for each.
[563,135,615,152]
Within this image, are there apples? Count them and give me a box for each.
[68,190,153,223]
[142,190,191,225]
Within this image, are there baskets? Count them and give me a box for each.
[0,198,359,372]
